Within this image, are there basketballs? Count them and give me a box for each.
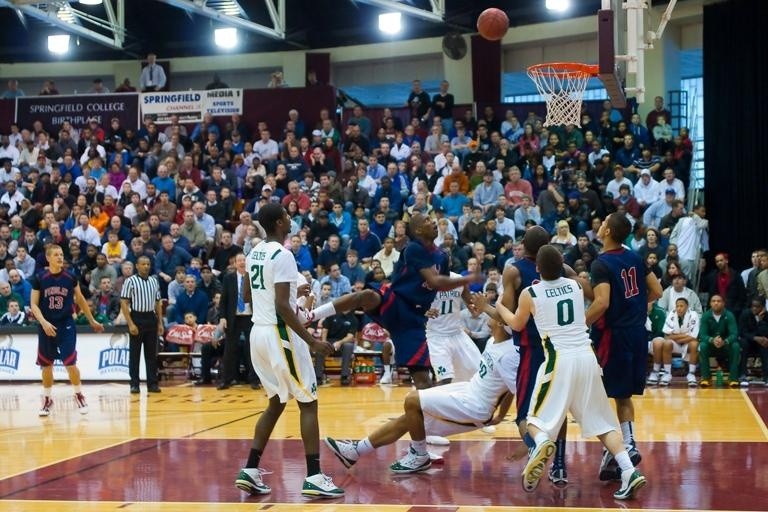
[475,8,508,42]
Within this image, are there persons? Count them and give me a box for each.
[0,79,25,98]
[121,257,161,392]
[307,70,323,87]
[495,246,646,498]
[204,71,231,90]
[585,213,663,481]
[114,77,136,93]
[422,270,495,431]
[403,79,432,131]
[234,202,346,499]
[39,79,59,96]
[140,53,167,93]
[86,79,110,94]
[325,318,520,472]
[299,212,484,444]
[1,96,768,390]
[470,226,577,485]
[430,80,454,135]
[266,70,289,89]
[31,245,104,416]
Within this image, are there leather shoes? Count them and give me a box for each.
[130,378,259,393]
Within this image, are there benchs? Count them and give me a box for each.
[155,348,766,385]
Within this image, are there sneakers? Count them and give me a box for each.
[521,440,556,492]
[235,468,270,494]
[324,436,360,469]
[614,467,645,500]
[302,472,345,497]
[72,392,87,414]
[389,445,432,473]
[599,449,619,481]
[39,396,53,416]
[547,463,568,487]
[483,426,496,434]
[625,440,642,467]
[426,435,450,445]
[296,305,312,329]
[647,369,749,387]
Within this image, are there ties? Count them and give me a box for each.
[239,276,245,313]
